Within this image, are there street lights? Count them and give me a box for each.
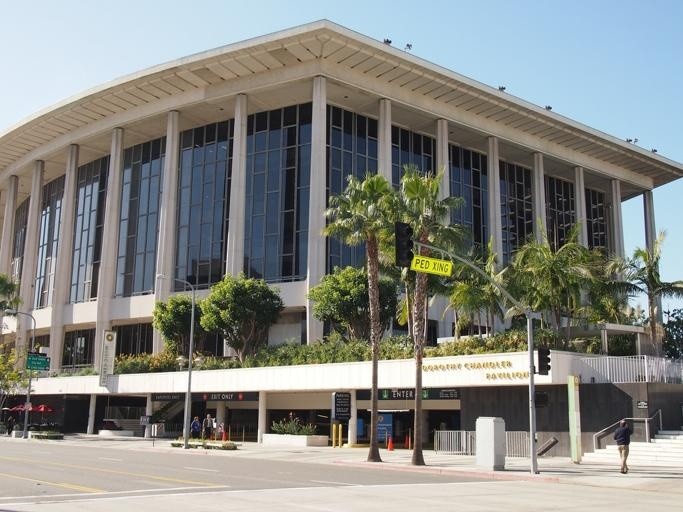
[154,272,195,450]
[5,307,35,439]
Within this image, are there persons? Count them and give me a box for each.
[190,416,202,438]
[8,415,15,435]
[203,413,213,439]
[614,420,631,473]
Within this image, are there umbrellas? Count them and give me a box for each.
[32,404,53,424]
[22,403,39,424]
[9,403,24,420]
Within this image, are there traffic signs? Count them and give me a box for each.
[27,354,50,371]
[410,257,451,277]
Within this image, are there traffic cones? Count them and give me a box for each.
[403,435,409,448]
[386,437,393,452]
[220,431,227,442]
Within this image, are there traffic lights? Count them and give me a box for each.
[538,346,552,375]
[395,223,413,266]
[535,390,546,406]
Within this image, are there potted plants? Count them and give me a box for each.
[45,413,63,440]
[98,421,134,437]
[304,422,329,445]
[260,411,308,448]
